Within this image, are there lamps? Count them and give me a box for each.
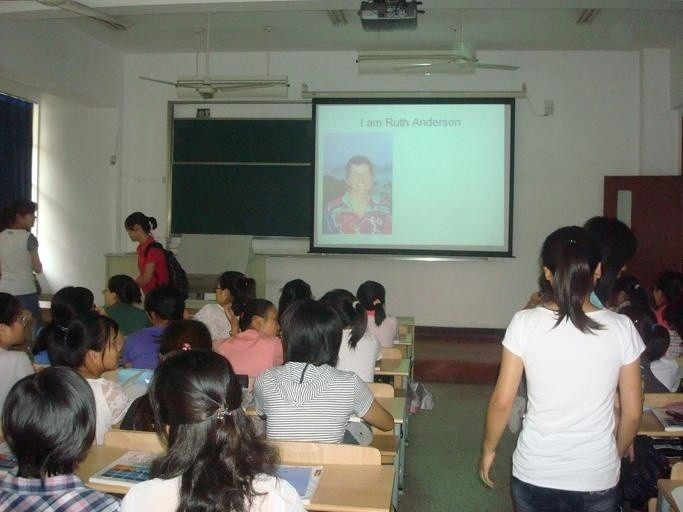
[357,25,475,74]
[177,26,289,98]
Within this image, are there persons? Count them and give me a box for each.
[0,200,42,331]
[103,275,305,512]
[0,285,130,512]
[326,156,391,235]
[480,224,648,511]
[527,216,637,506]
[126,211,184,318]
[195,271,398,444]
[610,269,683,507]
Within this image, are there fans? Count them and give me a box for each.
[138,12,274,100]
[392,9,520,75]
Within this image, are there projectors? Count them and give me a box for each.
[360,3,418,29]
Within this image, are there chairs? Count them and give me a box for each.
[186,273,219,300]
[378,347,404,390]
[101,431,168,455]
[394,325,408,359]
[258,440,381,466]
[363,383,395,436]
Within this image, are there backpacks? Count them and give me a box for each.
[619,435,671,512]
[144,241,189,300]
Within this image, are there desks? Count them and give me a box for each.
[70,443,396,511]
[613,392,683,512]
[374,359,412,447]
[35,363,408,507]
[395,333,413,395]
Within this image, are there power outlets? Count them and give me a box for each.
[544,100,554,116]
